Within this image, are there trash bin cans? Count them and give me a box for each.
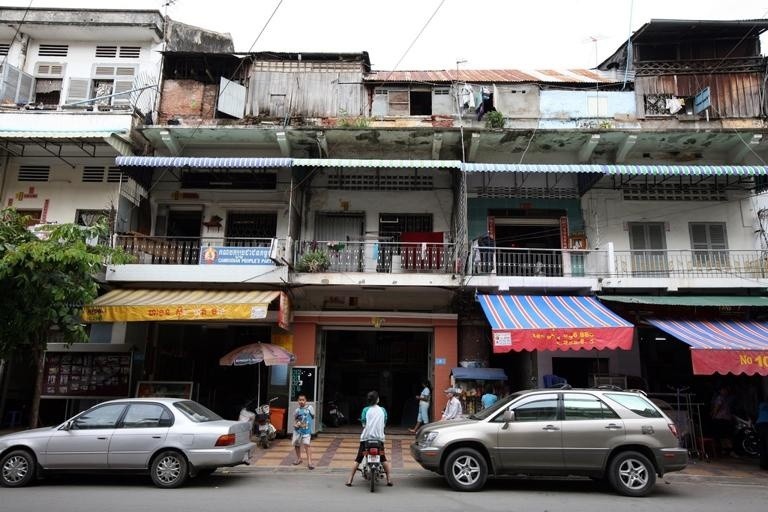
[270,407,286,432]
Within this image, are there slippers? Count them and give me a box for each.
[345,481,351,486]
[387,482,392,486]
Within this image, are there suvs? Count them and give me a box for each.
[409,384,689,496]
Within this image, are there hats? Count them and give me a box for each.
[443,387,456,393]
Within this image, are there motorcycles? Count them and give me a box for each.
[323,392,346,427]
[733,416,760,455]
[356,419,387,492]
[245,397,279,448]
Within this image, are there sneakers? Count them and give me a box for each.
[294,459,303,464]
[308,463,314,469]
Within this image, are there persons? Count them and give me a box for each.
[344,390,393,487]
[440,387,463,422]
[754,377,768,471]
[481,384,498,410]
[408,379,432,432]
[710,383,743,460]
[291,393,316,470]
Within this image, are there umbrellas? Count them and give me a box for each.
[219,340,296,410]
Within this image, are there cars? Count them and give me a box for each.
[0,397,257,488]
